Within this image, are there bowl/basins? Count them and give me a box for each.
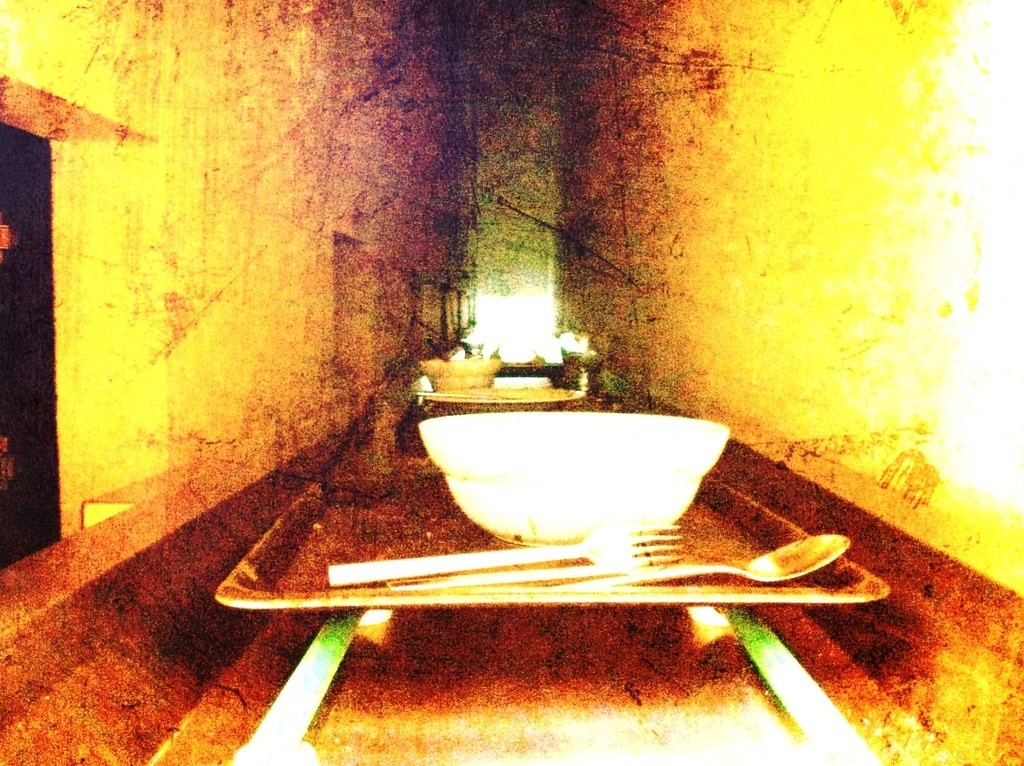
[420,413,733,545]
[416,358,501,391]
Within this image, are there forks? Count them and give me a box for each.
[325,523,686,590]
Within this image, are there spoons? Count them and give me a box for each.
[388,534,851,582]
